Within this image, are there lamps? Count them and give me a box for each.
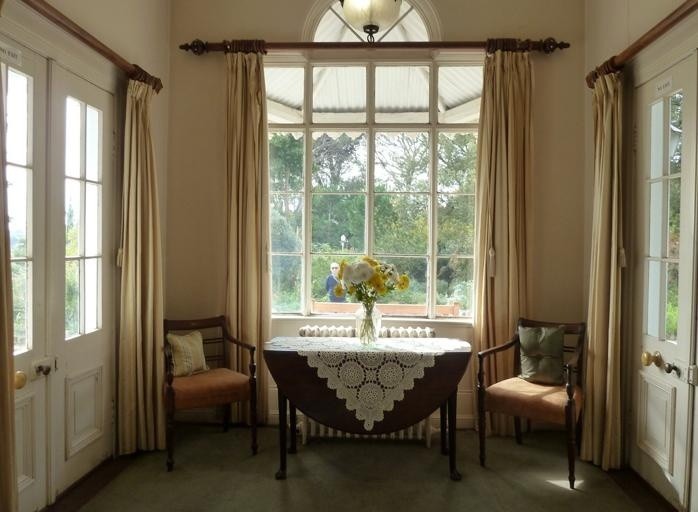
[338,0,402,41]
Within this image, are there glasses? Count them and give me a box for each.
[332,267,338,269]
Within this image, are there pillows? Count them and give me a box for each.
[167,329,209,377]
[516,326,568,386]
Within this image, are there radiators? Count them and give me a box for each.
[297,325,437,449]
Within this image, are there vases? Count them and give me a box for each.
[354,301,383,348]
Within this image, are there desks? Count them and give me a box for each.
[263,336,472,481]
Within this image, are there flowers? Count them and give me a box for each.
[336,255,409,339]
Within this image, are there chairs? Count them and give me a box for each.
[159,314,258,472]
[477,317,589,490]
